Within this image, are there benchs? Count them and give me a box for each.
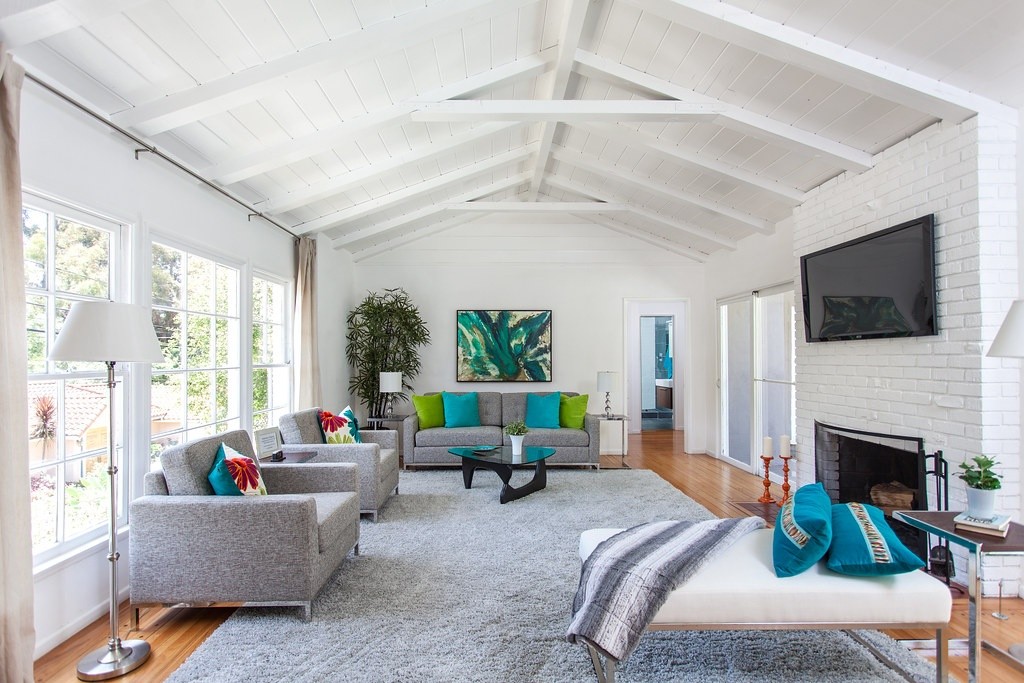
[566,517,952,683]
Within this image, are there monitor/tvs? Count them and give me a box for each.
[799,212,939,343]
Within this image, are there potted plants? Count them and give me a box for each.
[952,453,1003,519]
[505,415,529,454]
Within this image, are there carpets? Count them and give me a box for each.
[167,468,958,683]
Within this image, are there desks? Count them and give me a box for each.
[892,509,1024,683]
[367,414,409,430]
[593,414,629,455]
[262,451,317,463]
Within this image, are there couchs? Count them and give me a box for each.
[129,429,360,632]
[279,407,400,523]
[403,391,600,472]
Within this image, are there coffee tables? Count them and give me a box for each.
[448,446,557,504]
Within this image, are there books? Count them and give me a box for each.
[952,511,1011,538]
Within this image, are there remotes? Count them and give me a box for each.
[473,451,487,456]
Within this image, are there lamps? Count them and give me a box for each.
[48,301,166,682]
[380,371,402,417]
[986,300,1024,663]
[597,371,618,417]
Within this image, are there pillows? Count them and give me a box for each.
[411,390,589,429]
[209,441,267,496]
[773,483,926,577]
[317,406,360,444]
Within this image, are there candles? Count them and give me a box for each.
[761,434,790,457]
